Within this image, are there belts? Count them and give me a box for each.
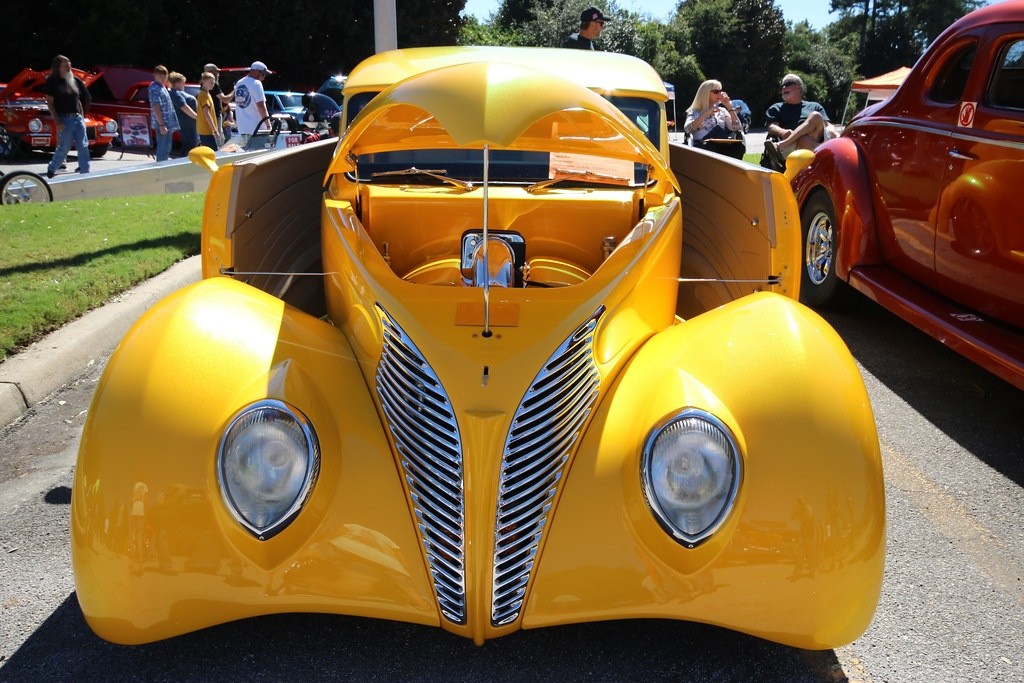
[63,112,79,118]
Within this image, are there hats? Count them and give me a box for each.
[251,61,272,75]
[204,63,221,72]
[579,6,611,21]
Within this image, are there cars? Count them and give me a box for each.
[88,66,347,148]
[791,1,1024,391]
[0,67,119,159]
[667,120,674,131]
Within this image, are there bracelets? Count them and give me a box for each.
[268,126,270,128]
[213,131,217,132]
[160,124,166,126]
[728,109,735,112]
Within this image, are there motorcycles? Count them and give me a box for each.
[0,170,53,207]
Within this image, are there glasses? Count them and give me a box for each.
[259,70,266,77]
[780,82,797,89]
[710,89,724,94]
[594,20,604,27]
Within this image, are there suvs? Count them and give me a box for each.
[730,99,752,134]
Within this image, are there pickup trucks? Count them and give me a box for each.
[71,44,886,652]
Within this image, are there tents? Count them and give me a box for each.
[841,66,912,133]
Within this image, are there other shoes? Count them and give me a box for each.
[48,167,54,178]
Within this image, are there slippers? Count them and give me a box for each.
[764,141,784,161]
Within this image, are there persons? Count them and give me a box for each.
[765,73,837,172]
[562,6,611,51]
[148,61,341,164]
[683,79,744,159]
[44,55,92,178]
